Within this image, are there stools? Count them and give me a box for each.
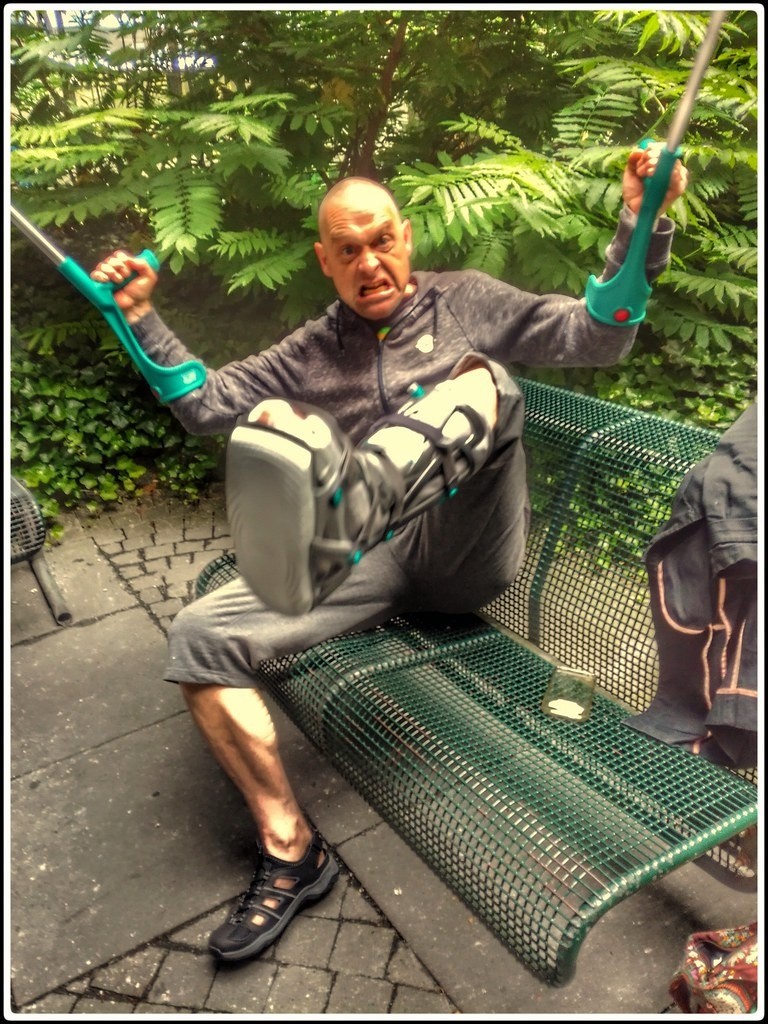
[10,475,73,627]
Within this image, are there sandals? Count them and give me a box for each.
[209,826,341,960]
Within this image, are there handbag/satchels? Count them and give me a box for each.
[670,920,757,1013]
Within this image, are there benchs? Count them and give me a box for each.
[194,373,758,989]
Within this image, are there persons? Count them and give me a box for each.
[89,142,690,960]
